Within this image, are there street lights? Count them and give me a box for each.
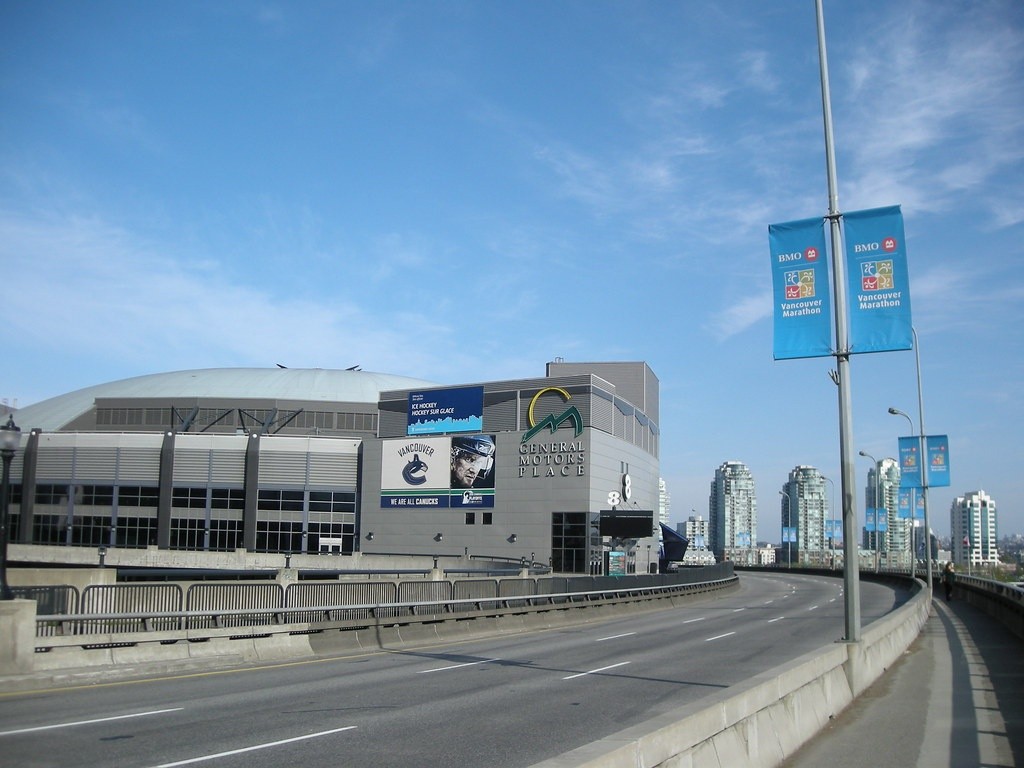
[692,509,700,566]
[889,406,916,580]
[736,500,746,567]
[0,414,22,600]
[820,475,835,574]
[778,489,791,570]
[859,451,880,578]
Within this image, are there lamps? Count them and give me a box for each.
[303,531,307,537]
[511,533,517,542]
[205,528,209,535]
[111,524,116,532]
[67,523,71,530]
[369,532,373,539]
[437,533,442,541]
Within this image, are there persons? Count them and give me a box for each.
[940,562,956,601]
[451,435,485,489]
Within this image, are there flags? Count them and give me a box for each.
[963,536,970,546]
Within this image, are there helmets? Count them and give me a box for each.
[451,434,495,471]
